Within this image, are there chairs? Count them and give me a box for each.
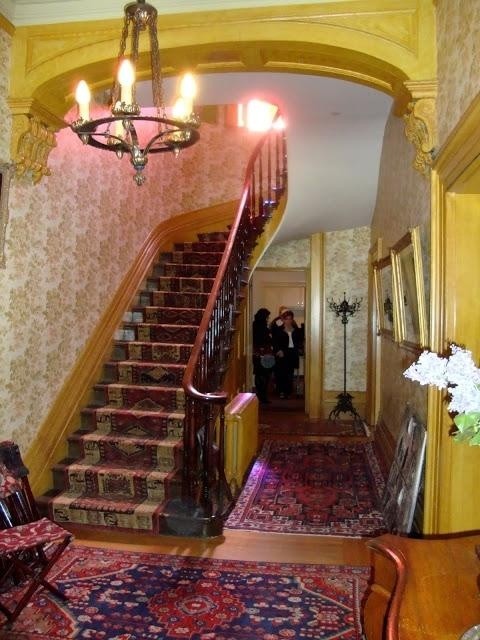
[0,517,70,628]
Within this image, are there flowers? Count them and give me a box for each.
[399,336,480,447]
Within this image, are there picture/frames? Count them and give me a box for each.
[370,226,427,353]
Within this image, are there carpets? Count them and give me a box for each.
[0,544,375,640]
[222,415,394,538]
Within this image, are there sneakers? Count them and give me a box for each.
[277,391,291,399]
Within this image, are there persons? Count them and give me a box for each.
[253,308,275,406]
[298,321,306,347]
[271,309,305,400]
[269,305,288,334]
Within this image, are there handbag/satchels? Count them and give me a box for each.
[260,354,276,368]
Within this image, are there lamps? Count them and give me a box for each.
[63,0,200,189]
